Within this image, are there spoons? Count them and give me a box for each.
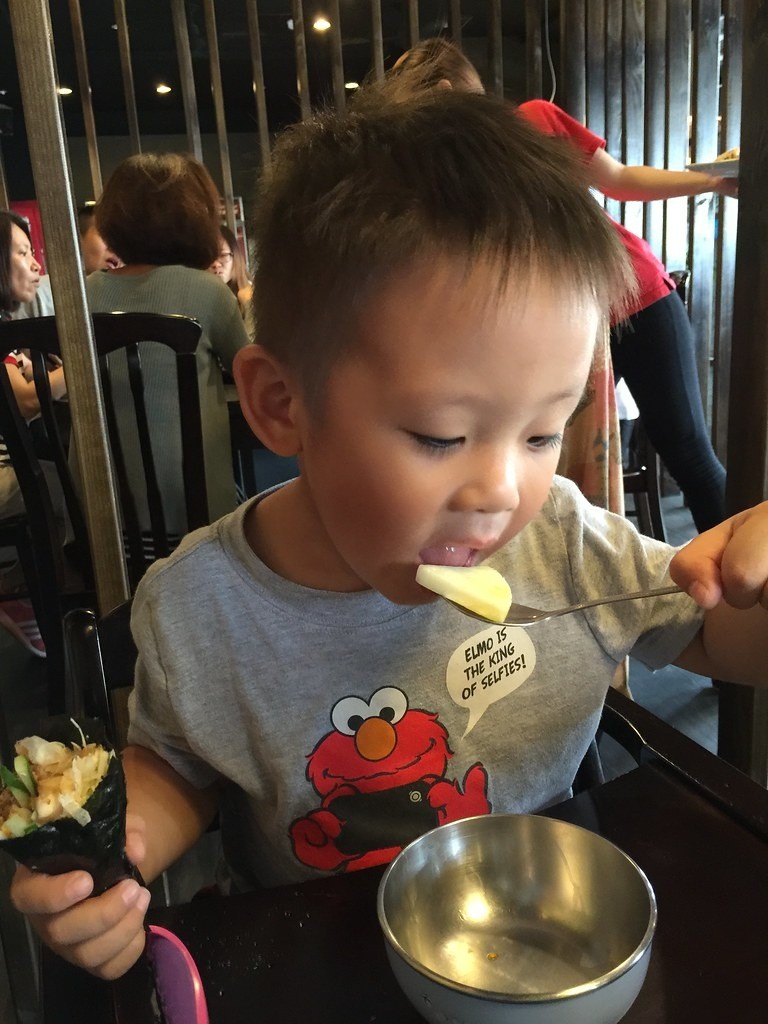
[438,584,686,627]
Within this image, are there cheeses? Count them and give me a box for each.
[415,564,513,624]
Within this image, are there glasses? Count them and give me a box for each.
[217,252,233,263]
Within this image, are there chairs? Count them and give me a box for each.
[624,270,691,542]
[0,311,211,748]
[0,588,768,1024]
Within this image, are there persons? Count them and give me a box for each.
[0,35,741,687]
[10,87,768,982]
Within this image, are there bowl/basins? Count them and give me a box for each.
[376,813,658,1024]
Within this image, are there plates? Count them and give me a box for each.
[685,159,739,177]
